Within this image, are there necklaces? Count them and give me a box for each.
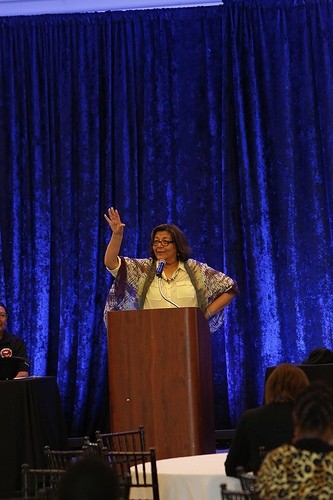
[161,269,180,281]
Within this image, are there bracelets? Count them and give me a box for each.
[205,308,212,321]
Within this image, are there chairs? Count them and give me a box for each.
[220,466,261,500]
[20,426,159,500]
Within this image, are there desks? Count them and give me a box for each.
[129,453,242,499]
[0,376,64,497]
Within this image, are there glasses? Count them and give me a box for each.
[1,312,8,318]
[152,240,176,247]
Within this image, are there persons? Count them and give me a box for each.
[56,457,120,500]
[0,301,30,379]
[103,206,240,335]
[224,347,333,499]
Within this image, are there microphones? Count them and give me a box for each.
[156,260,166,276]
